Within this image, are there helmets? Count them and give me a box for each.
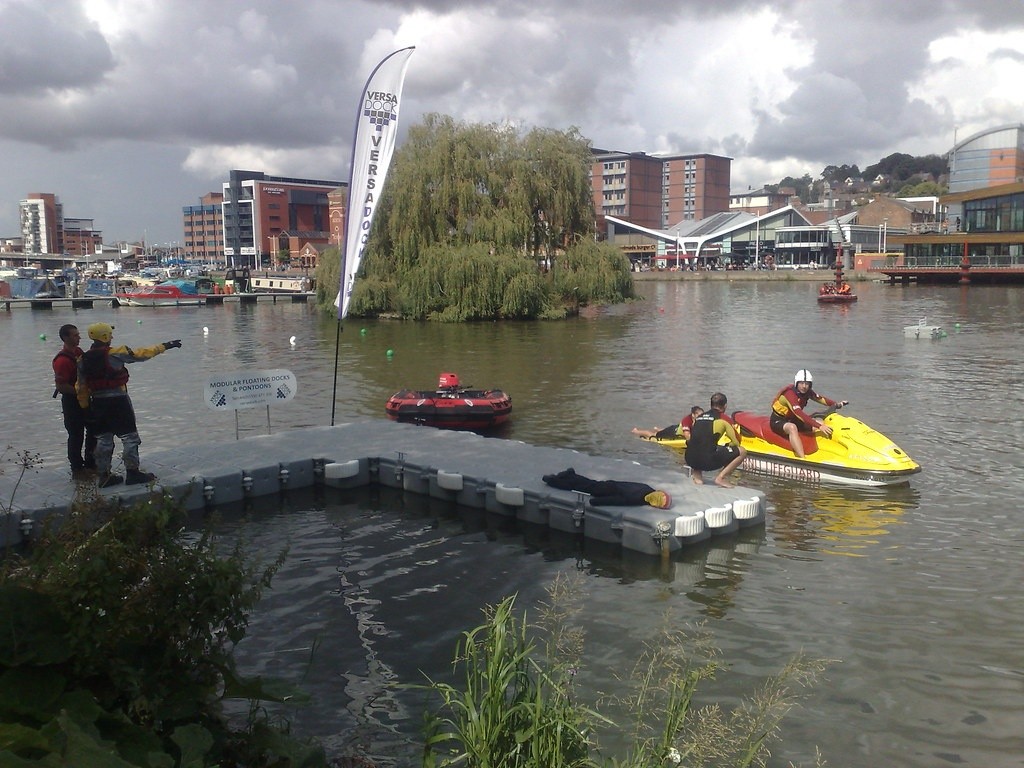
[828,282,833,286]
[794,369,813,388]
[842,282,846,284]
[87,322,113,343]
[823,282,828,285]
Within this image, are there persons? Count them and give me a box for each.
[809,260,818,270]
[684,392,747,487]
[74,322,181,487]
[52,324,97,466]
[943,216,961,234]
[632,406,705,441]
[770,369,843,458]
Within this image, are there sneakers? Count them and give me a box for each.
[99,472,123,488]
[126,469,154,485]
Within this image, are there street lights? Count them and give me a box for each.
[752,210,759,270]
[883,217,888,253]
[676,228,680,269]
[878,224,883,253]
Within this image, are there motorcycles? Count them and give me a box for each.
[641,400,922,486]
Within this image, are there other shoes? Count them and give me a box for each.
[84,464,97,470]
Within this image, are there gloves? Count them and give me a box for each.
[161,340,182,350]
[80,408,96,423]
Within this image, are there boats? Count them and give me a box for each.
[386,372,514,430]
[115,285,206,307]
[817,248,857,302]
[9,267,164,297]
